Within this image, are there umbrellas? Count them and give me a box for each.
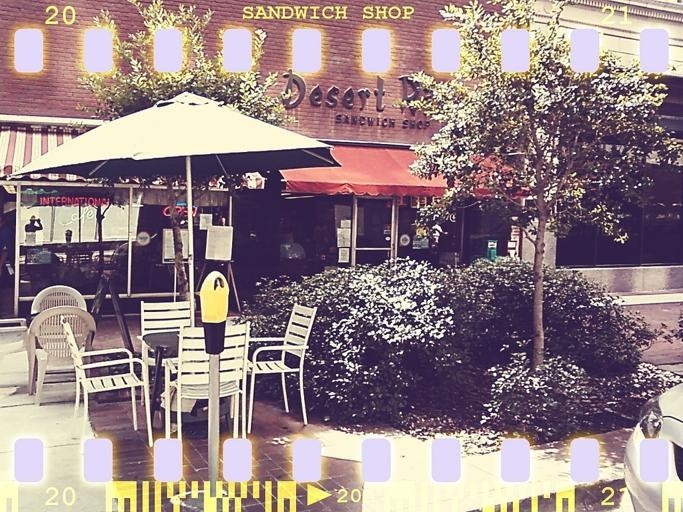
[9,90,342,328]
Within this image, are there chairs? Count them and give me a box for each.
[23,283,318,449]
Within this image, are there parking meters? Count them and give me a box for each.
[199,272,230,481]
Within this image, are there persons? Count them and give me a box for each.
[0,212,11,288]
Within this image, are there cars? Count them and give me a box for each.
[619,382,683,512]
